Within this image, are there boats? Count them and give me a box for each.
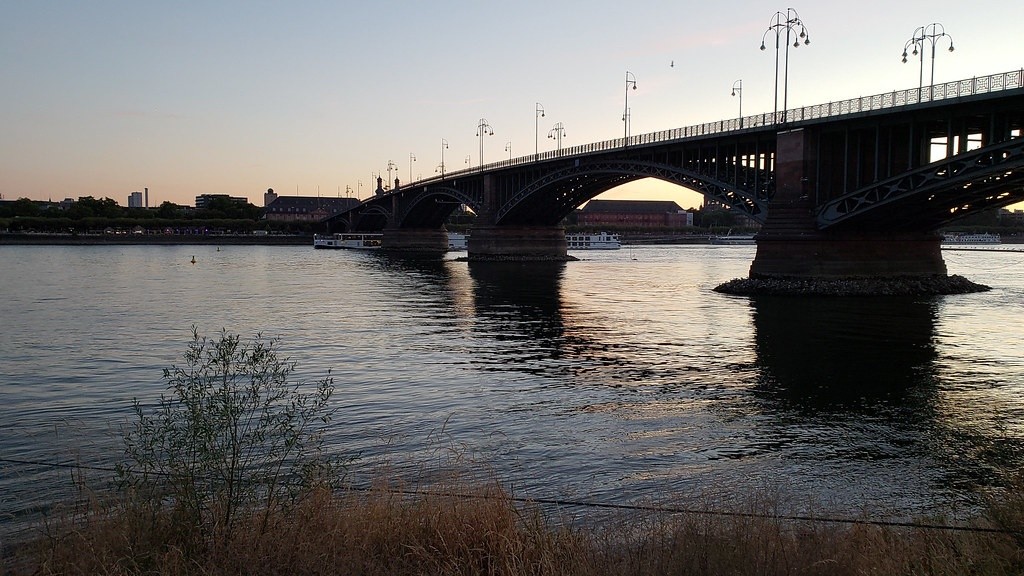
[313,232,470,248]
[565,231,623,249]
[941,232,1002,245]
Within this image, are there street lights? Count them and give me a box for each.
[357,179,362,199]
[441,138,448,179]
[761,7,812,124]
[386,160,398,190]
[476,117,494,170]
[372,171,377,196]
[548,122,566,152]
[505,142,512,164]
[465,154,472,170]
[535,101,545,155]
[625,70,637,144]
[899,21,954,102]
[622,107,633,140]
[731,79,744,122]
[345,185,354,209]
[409,152,417,184]
